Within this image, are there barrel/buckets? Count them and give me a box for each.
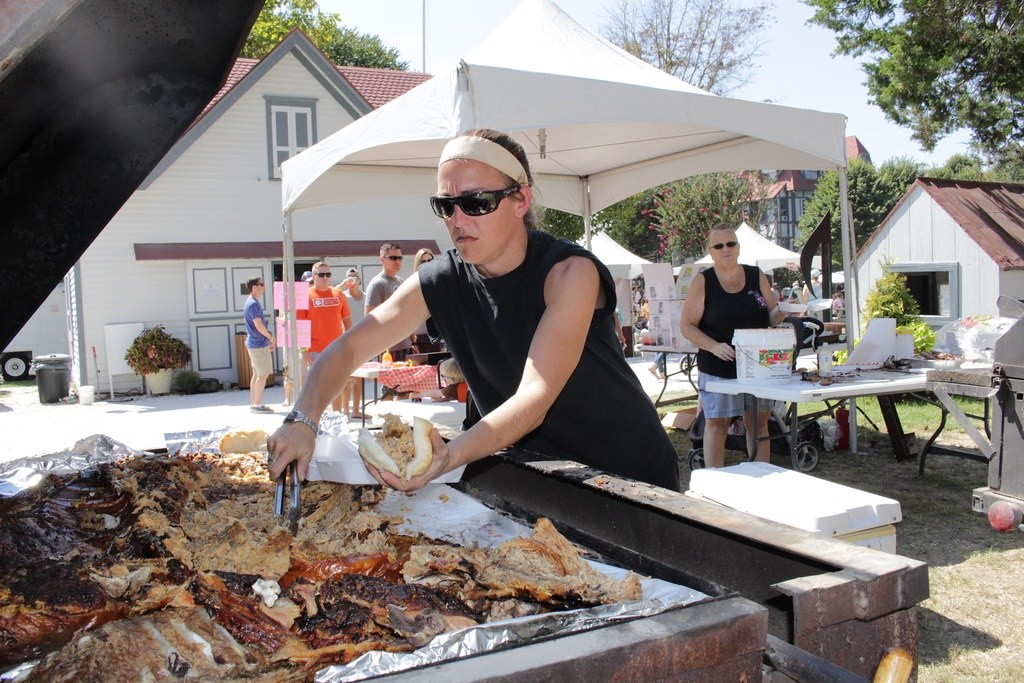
[731,328,796,385]
[78,386,94,404]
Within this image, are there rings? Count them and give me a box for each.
[268,455,275,462]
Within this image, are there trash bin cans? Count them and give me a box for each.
[34,353,73,404]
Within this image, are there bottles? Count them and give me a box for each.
[819,341,832,378]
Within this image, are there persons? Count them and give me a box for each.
[648,353,667,380]
[267,128,682,493]
[244,276,276,414]
[771,269,845,317]
[681,228,787,468]
[414,248,442,353]
[365,242,420,401]
[409,357,468,402]
[301,262,373,422]
[631,285,650,332]
[615,308,626,344]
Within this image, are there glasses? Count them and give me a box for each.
[315,273,331,278]
[254,283,263,287]
[386,255,403,261]
[429,185,520,220]
[709,242,739,250]
[421,259,433,263]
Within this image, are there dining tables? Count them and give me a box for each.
[350,362,437,429]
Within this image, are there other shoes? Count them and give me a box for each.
[250,404,274,414]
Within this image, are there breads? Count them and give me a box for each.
[217,428,269,453]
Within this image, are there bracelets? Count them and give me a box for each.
[269,336,274,342]
[412,342,418,347]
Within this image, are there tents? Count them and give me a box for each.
[673,221,822,276]
[570,231,653,358]
[279,0,858,453]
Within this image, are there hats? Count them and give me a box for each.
[300,271,313,282]
[346,268,362,285]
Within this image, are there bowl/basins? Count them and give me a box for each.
[777,298,834,312]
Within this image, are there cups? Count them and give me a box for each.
[345,277,356,287]
[641,328,657,345]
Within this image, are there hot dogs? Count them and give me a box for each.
[357,412,435,479]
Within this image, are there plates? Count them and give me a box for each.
[833,362,884,371]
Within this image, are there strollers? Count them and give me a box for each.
[686,316,825,473]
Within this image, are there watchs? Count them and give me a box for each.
[283,411,319,438]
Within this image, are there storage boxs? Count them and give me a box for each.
[685,462,901,553]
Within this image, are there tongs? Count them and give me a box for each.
[272,460,300,539]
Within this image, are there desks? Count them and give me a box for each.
[639,343,699,409]
[703,366,990,485]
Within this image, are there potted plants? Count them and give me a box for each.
[124,325,193,393]
